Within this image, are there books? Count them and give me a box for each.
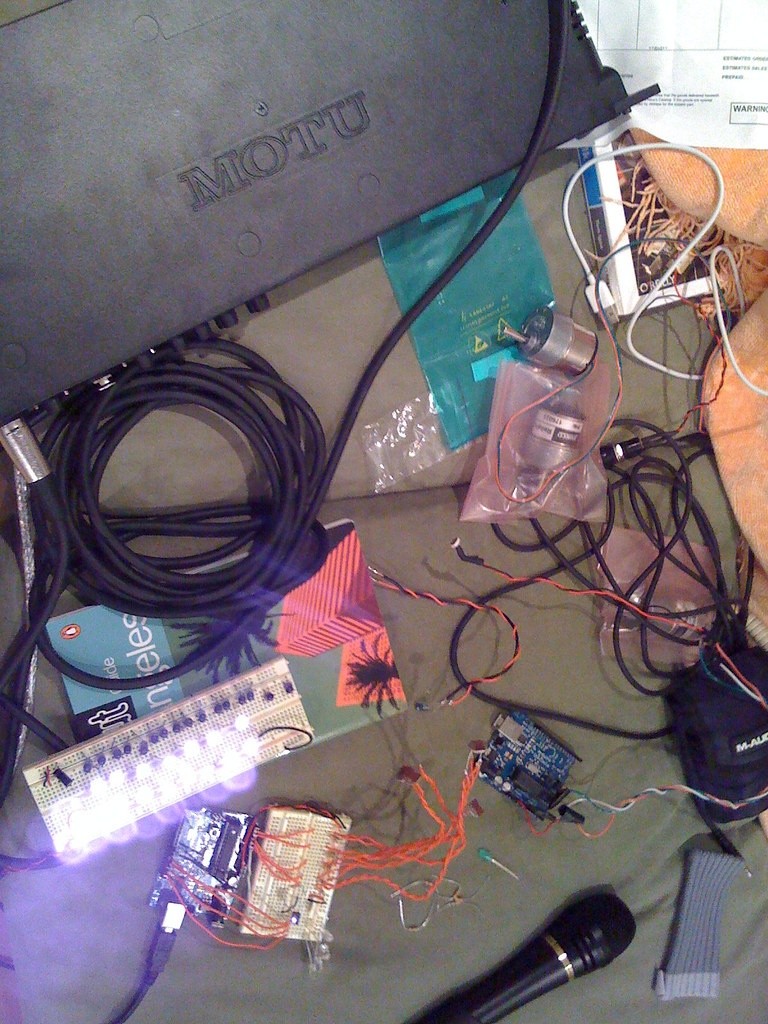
[578,143,722,317]
[46,519,409,758]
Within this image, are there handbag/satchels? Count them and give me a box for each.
[665,647,768,822]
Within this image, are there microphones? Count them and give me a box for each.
[405,892,637,1024]
